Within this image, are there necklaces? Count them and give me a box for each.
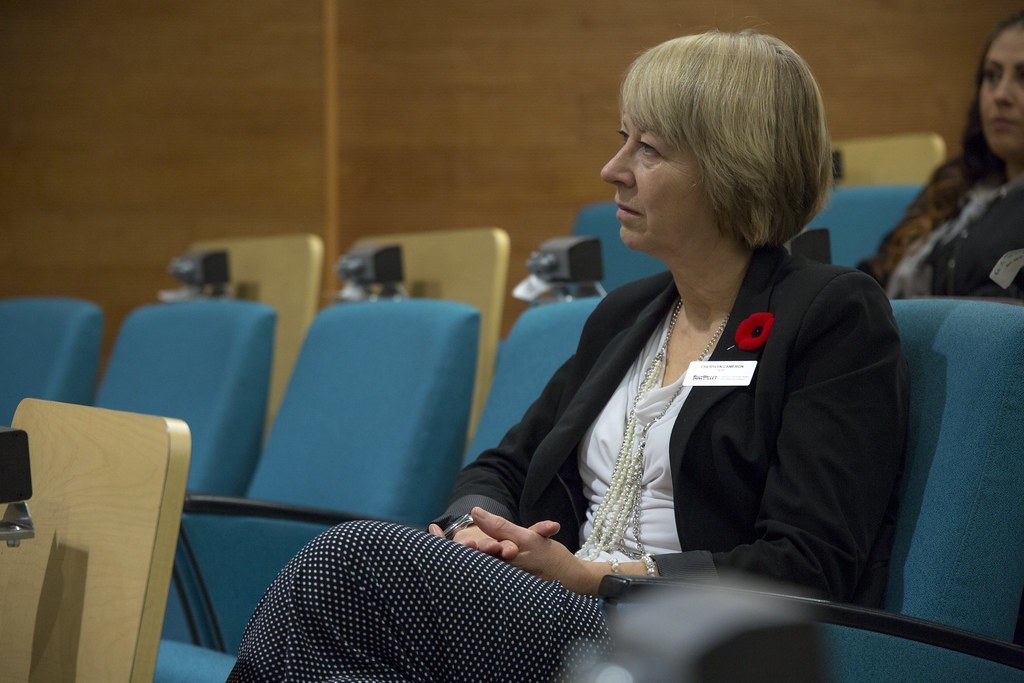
[575,295,732,562]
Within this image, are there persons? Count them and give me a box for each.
[220,26,908,682]
[853,11,1023,307]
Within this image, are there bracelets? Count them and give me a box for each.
[609,554,656,580]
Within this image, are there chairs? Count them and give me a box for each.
[458,301,603,467]
[190,236,324,441]
[150,299,484,683]
[0,296,103,425]
[808,184,926,268]
[0,398,191,682]
[94,297,280,501]
[358,226,510,456]
[561,201,669,295]
[596,299,1024,683]
